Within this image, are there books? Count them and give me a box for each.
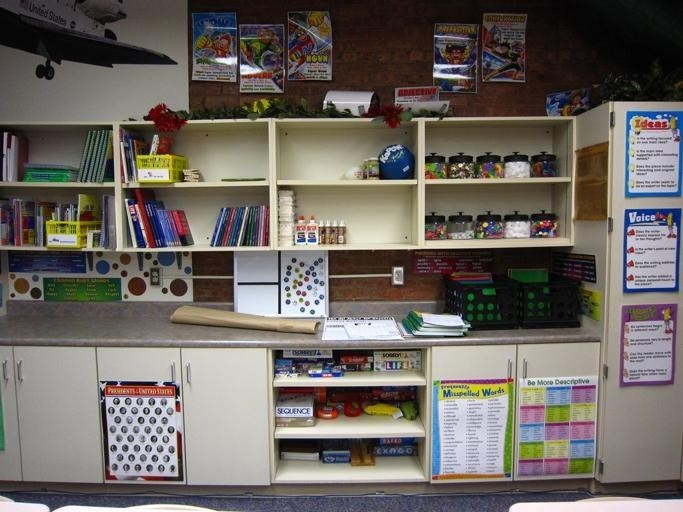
[0,129,270,247]
[447,264,554,329]
[395,311,474,339]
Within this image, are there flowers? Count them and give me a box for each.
[141,98,444,135]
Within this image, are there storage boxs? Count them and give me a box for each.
[135,151,187,183]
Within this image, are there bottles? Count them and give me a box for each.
[293,214,345,244]
[425,152,557,178]
[363,157,380,179]
[425,210,558,239]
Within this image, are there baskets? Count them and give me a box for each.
[443,274,581,330]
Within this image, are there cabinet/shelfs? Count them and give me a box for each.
[431,346,595,492]
[575,100,683,484]
[0,346,104,485]
[96,345,271,490]
[0,115,576,251]
[270,349,430,484]
